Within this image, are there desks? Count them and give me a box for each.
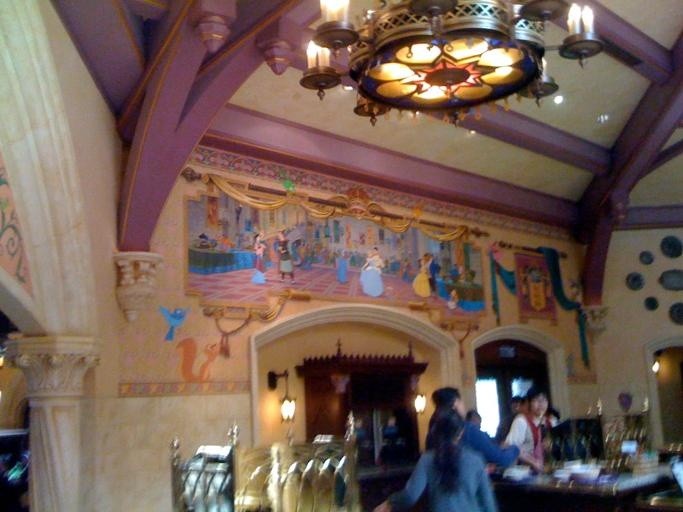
[491,460,677,510]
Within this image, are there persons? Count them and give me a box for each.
[373,383,560,511]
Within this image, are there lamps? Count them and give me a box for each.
[299,1,604,127]
[267,369,297,423]
[411,364,428,415]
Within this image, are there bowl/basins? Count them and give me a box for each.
[568,469,599,483]
